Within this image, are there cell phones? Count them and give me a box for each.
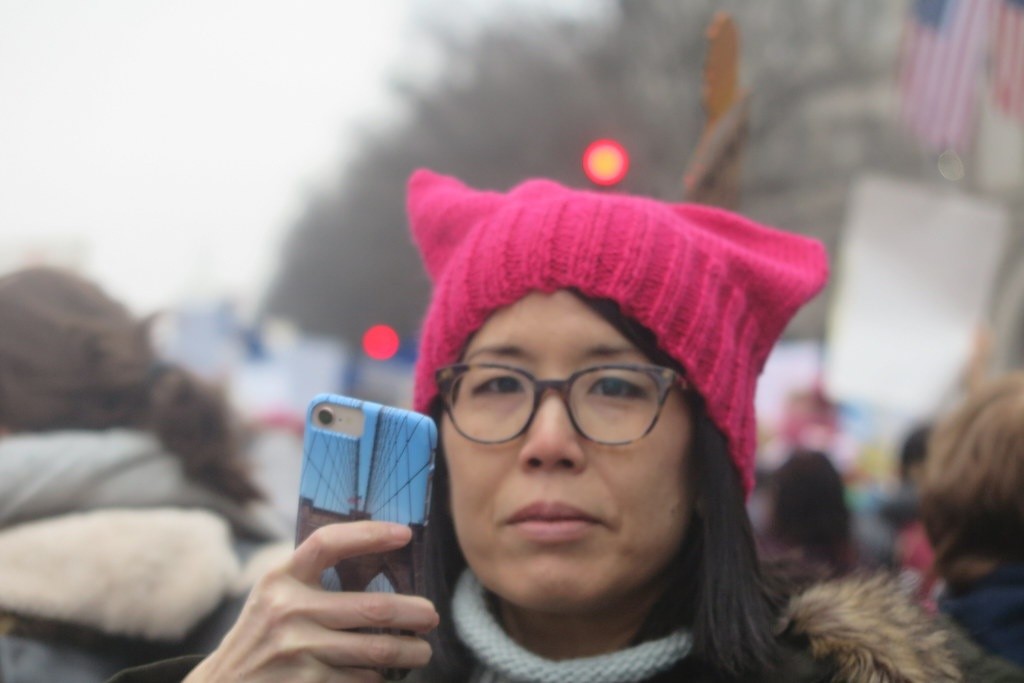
[292,393,437,680]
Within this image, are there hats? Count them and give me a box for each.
[407,168,827,505]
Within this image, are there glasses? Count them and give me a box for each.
[434,360,703,445]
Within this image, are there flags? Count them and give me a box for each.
[887,0,1024,156]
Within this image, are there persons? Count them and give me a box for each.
[754,371,1024,683]
[110,169,1024,683]
[0,264,289,683]
